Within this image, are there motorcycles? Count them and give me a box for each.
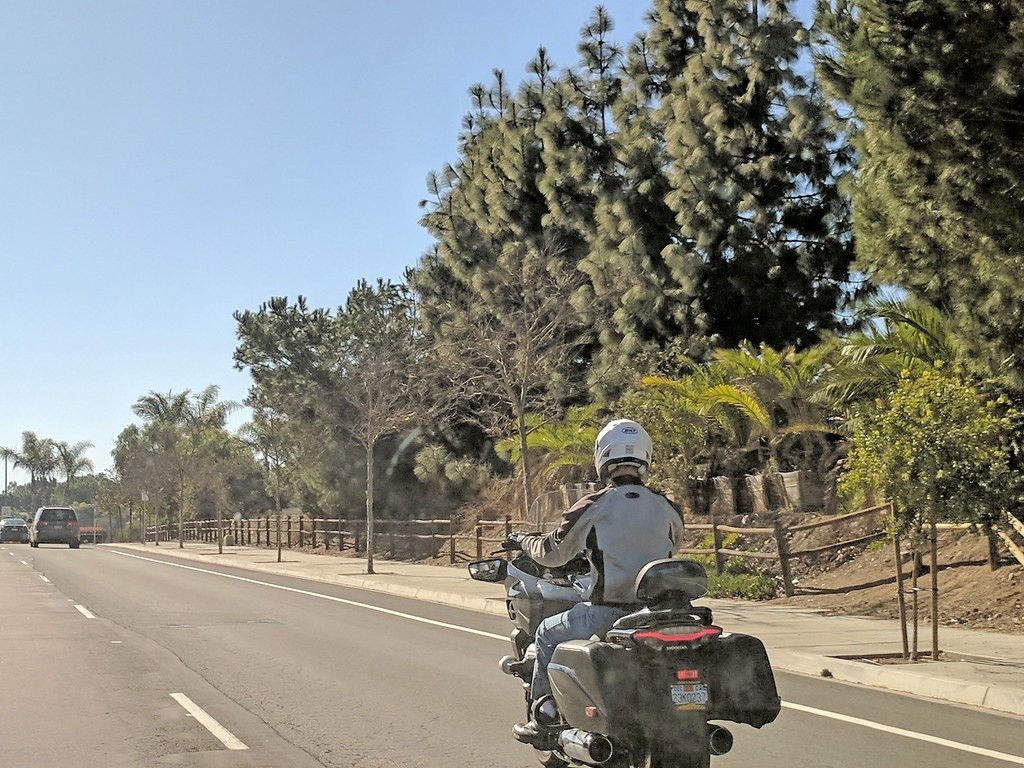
[467,489,782,768]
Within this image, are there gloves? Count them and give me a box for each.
[507,530,541,545]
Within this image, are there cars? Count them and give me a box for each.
[0,518,29,544]
[27,506,80,548]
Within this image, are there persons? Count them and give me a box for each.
[512,419,685,750]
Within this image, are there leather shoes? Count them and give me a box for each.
[513,722,552,751]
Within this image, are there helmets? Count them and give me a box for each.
[594,419,654,481]
